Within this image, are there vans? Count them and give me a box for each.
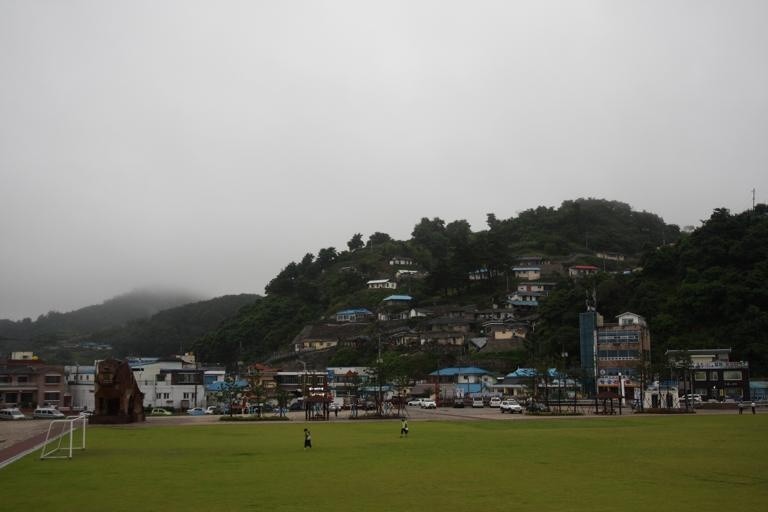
[0,407,25,420]
[33,408,64,419]
[472,396,483,408]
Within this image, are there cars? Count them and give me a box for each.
[186,406,206,415]
[151,408,172,416]
[453,398,464,408]
[407,397,436,409]
[205,405,216,415]
[525,402,551,412]
[328,402,342,412]
[499,401,521,414]
[80,411,93,418]
[678,393,701,403]
[488,396,501,408]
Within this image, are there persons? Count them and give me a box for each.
[737,402,744,416]
[334,403,338,416]
[400,416,408,439]
[751,400,756,414]
[242,405,258,414]
[303,428,311,449]
[381,402,395,416]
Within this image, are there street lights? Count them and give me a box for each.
[688,363,693,408]
